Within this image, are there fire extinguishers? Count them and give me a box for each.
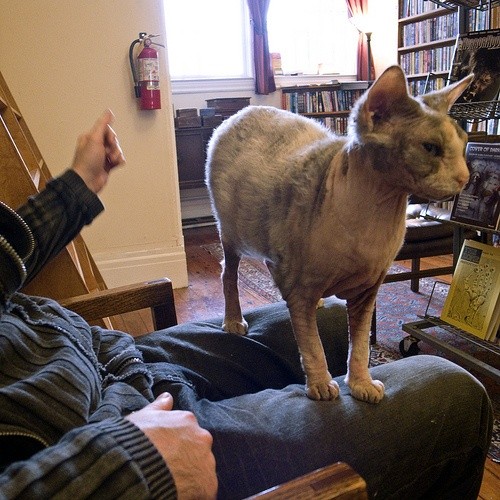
[128,32,166,113]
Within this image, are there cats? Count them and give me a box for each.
[205,65,475,406]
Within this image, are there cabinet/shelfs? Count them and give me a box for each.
[0,69,118,333]
[280,80,376,137]
[394,0,500,284]
[205,97,251,121]
[174,127,216,189]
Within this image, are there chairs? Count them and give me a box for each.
[371,204,480,344]
[52,278,368,499]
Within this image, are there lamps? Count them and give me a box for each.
[352,11,382,35]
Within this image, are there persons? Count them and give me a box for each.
[0,108,494,500]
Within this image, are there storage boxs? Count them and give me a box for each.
[174,108,223,129]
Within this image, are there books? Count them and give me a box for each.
[447,28,500,103]
[450,141,500,231]
[283,0,488,135]
[440,239,500,342]
